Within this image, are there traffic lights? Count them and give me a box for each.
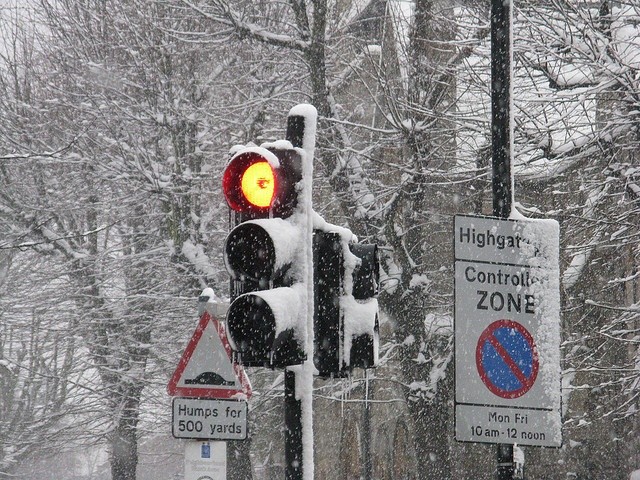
[222,147,308,372]
[313,229,380,380]
